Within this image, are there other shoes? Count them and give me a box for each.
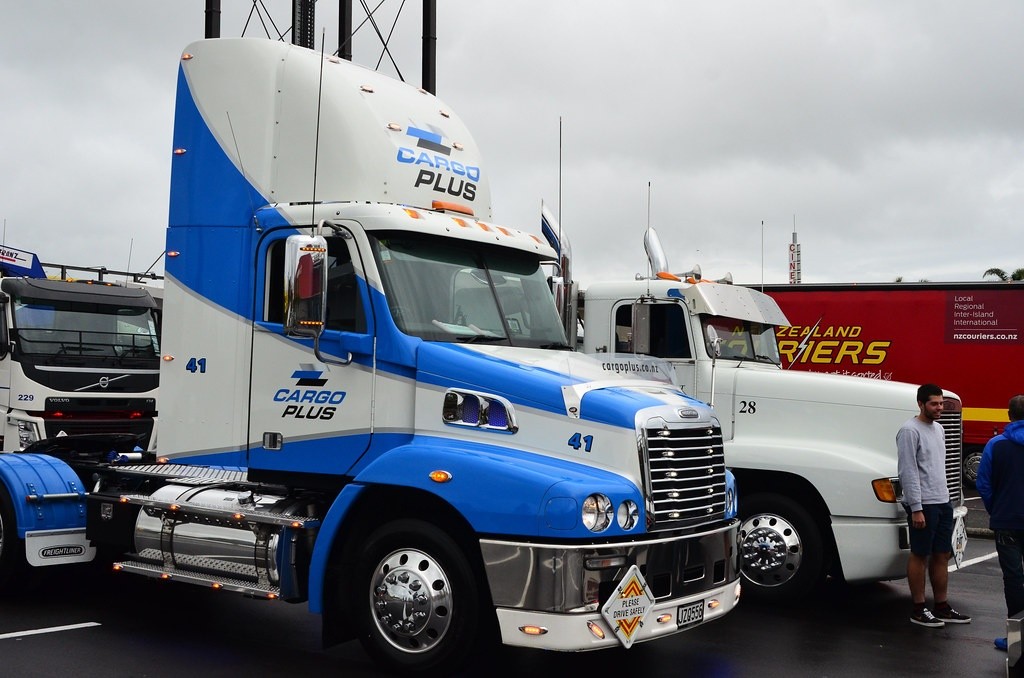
[932,605,971,623]
[910,608,945,627]
[995,637,1007,650]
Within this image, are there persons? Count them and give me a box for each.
[976,394,1024,650]
[895,382,971,628]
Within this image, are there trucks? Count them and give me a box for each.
[0,38,743,677]
[451,192,970,612]
[0,243,163,454]
